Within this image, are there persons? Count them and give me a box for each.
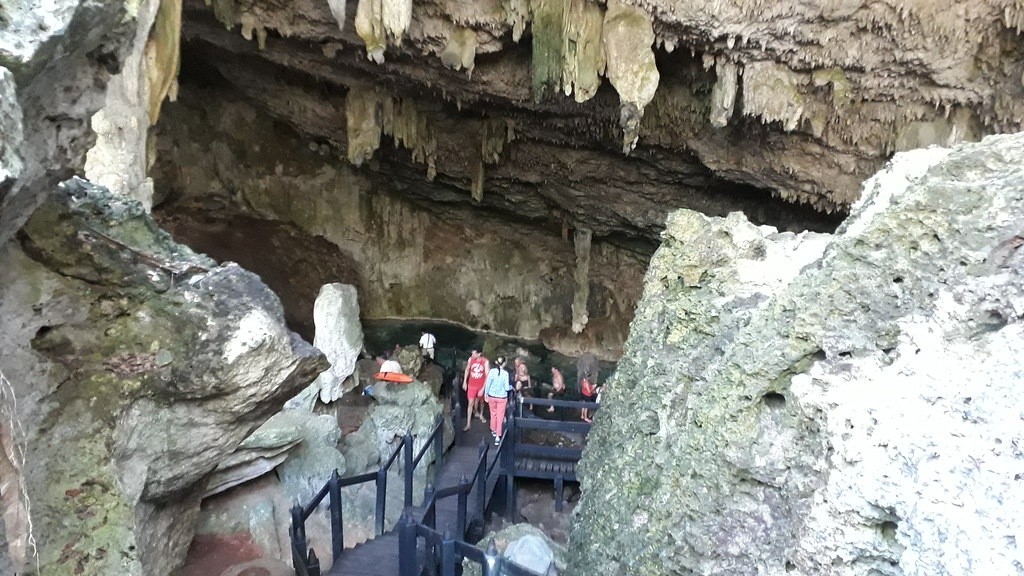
[591,383,606,420]
[547,364,568,412]
[580,371,597,423]
[485,355,515,446]
[473,396,488,423]
[462,347,490,432]
[375,352,403,374]
[418,329,436,359]
[503,357,534,424]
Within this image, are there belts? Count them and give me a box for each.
[488,395,507,399]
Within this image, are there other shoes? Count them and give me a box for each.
[462,425,472,431]
[480,416,487,423]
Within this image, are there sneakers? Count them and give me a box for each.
[495,437,500,445]
[491,431,496,437]
